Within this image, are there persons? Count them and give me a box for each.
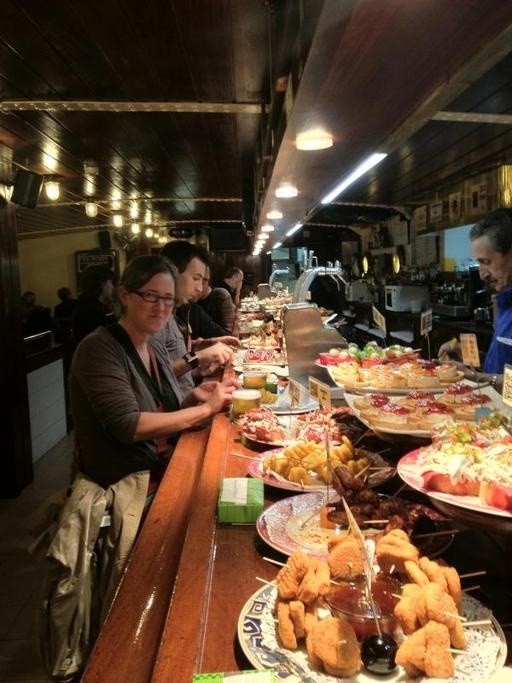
[19,292,41,324]
[195,270,235,335]
[436,205,511,394]
[63,266,114,496]
[217,267,243,305]
[68,254,241,515]
[55,288,76,320]
[173,301,225,340]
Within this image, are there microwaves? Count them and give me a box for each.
[383,284,430,313]
[344,281,374,303]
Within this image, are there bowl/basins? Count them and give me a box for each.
[319,572,411,640]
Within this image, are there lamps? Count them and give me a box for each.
[294,128,333,151]
[112,210,155,238]
[84,196,98,217]
[43,175,61,200]
[276,183,298,198]
[252,210,284,255]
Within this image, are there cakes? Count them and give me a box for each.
[320,341,512,512]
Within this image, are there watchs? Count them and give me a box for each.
[184,352,199,369]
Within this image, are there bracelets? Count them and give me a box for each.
[490,372,498,385]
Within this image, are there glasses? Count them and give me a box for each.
[119,283,179,306]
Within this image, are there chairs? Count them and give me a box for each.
[39,486,116,682]
[70,437,160,509]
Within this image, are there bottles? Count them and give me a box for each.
[408,261,444,282]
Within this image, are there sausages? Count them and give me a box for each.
[326,466,446,539]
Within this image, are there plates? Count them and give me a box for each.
[242,414,357,447]
[230,281,323,421]
[245,446,398,494]
[326,365,489,395]
[344,384,512,439]
[256,490,455,562]
[237,575,508,682]
[396,442,512,519]
[315,356,402,371]
